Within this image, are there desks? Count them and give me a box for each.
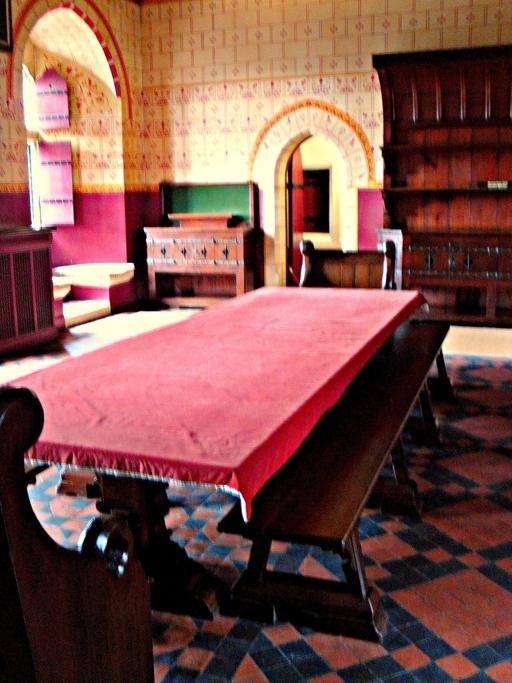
[0,285,428,620]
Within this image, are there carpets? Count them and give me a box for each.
[0,331,512,683]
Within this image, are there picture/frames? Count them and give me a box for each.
[0,0,14,51]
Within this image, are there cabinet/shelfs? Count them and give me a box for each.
[143,214,254,308]
[371,43,512,329]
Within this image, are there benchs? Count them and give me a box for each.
[52,277,72,332]
[52,262,135,309]
[216,318,451,642]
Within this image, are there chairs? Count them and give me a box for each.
[1,386,155,683]
[297,238,400,290]
